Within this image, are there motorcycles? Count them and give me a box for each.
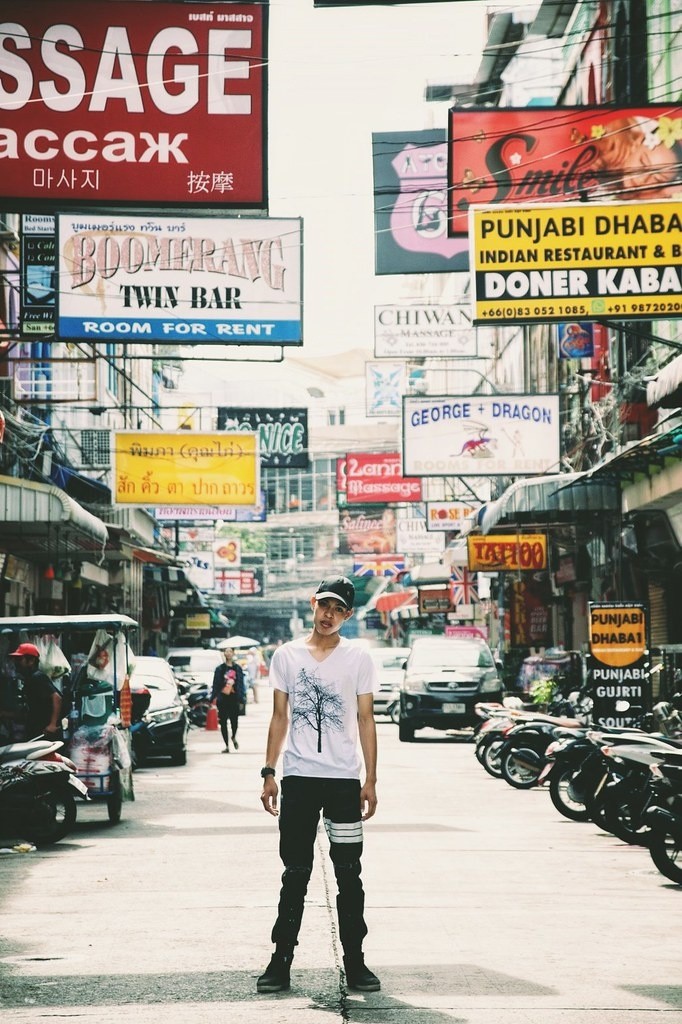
[174,675,216,728]
[473,688,682,888]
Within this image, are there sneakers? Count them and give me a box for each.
[255,952,292,993]
[343,952,381,991]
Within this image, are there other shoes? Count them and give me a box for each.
[222,749,230,753]
[231,736,239,750]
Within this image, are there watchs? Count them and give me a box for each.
[260,767,275,777]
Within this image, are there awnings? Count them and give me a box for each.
[447,470,627,568]
[0,475,112,565]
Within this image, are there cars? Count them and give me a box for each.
[166,649,246,714]
[397,636,505,741]
[372,648,411,725]
[129,657,192,766]
[234,645,275,678]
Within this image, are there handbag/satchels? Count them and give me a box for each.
[205,705,219,730]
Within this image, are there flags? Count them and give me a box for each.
[448,563,480,605]
[351,551,406,577]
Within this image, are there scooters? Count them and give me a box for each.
[1,740,91,847]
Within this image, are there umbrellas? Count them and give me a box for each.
[214,633,260,650]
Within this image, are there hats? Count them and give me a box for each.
[316,574,357,610]
[9,642,39,658]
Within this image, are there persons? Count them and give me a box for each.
[10,643,67,758]
[210,647,244,754]
[246,647,260,705]
[257,572,379,997]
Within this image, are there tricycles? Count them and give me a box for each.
[0,614,139,823]
